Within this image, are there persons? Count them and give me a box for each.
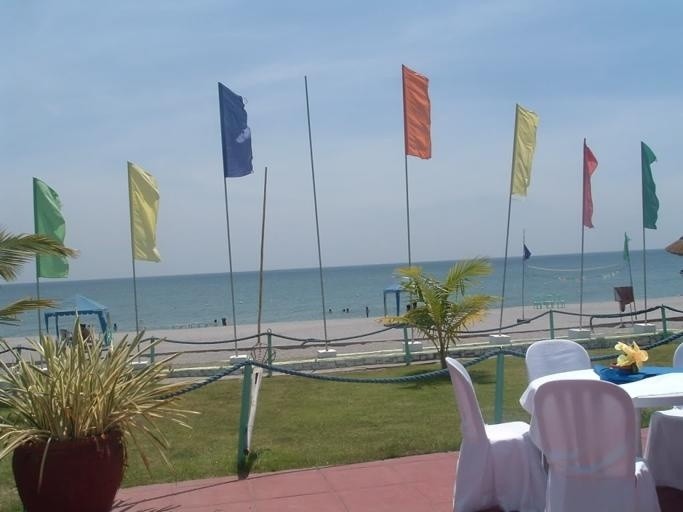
[80,324,90,341]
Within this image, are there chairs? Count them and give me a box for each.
[556,293,565,309]
[528,379,661,512]
[544,294,553,309]
[532,296,543,309]
[645,342,683,490]
[526,339,591,381]
[444,357,547,512]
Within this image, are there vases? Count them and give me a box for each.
[612,363,633,370]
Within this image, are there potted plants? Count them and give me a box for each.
[0,317,184,512]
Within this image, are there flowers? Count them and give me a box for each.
[614,340,649,372]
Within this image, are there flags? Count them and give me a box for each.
[33,177,69,277]
[402,64,432,159]
[523,244,532,260]
[581,138,598,229]
[623,233,631,260]
[641,141,659,229]
[510,102,537,196]
[127,161,161,263]
[218,81,255,177]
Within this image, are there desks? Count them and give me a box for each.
[519,369,683,456]
